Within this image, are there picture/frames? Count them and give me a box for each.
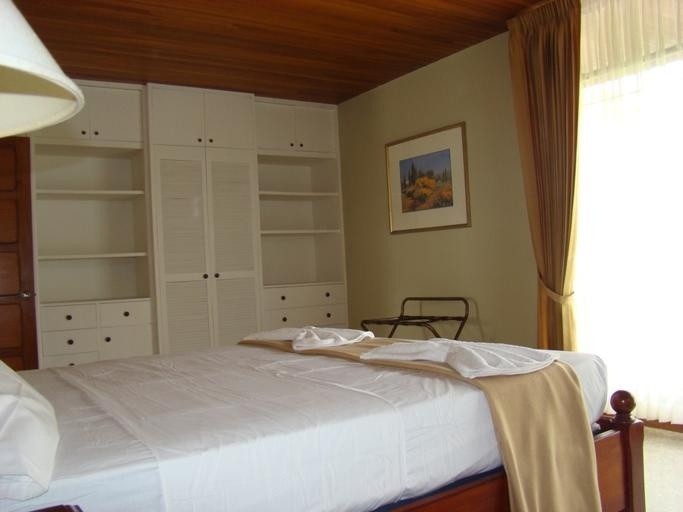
[383,119,471,233]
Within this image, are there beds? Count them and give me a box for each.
[1,332,648,511]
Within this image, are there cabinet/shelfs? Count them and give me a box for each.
[31,143,157,303]
[253,101,337,154]
[150,143,261,350]
[149,85,254,150]
[30,82,143,146]
[260,284,346,329]
[256,150,347,287]
[35,301,154,368]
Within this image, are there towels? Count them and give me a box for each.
[241,325,378,352]
[356,335,557,380]
